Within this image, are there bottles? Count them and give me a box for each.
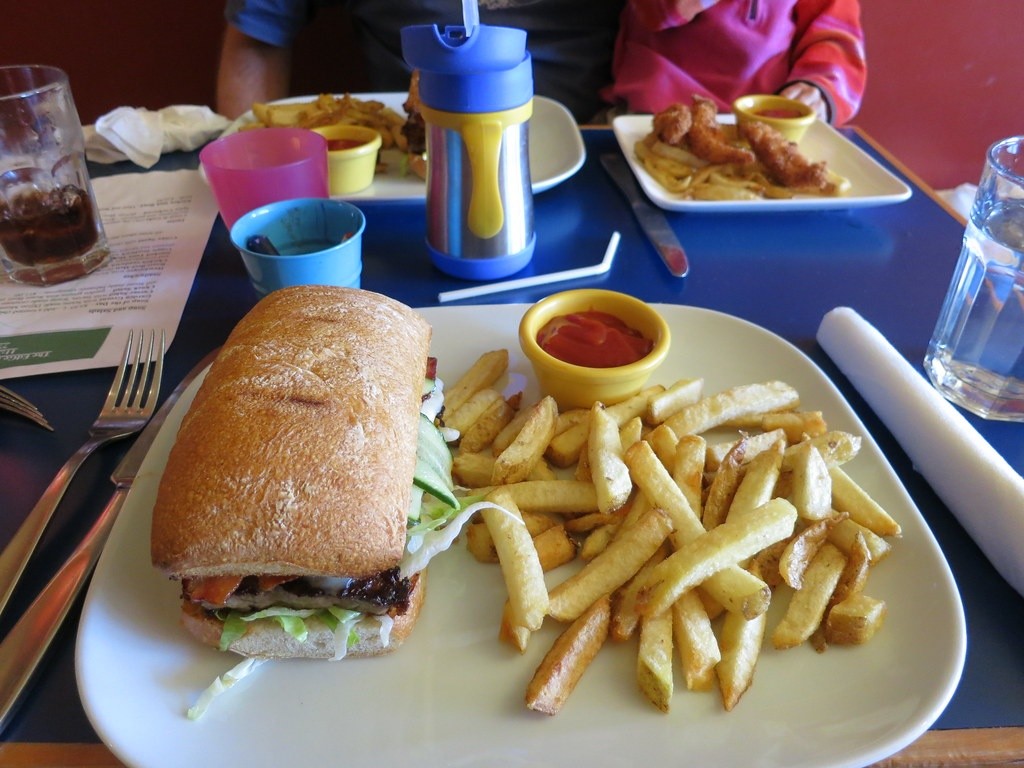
[399,1,537,280]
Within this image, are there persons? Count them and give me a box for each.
[216,0,627,126]
[587,0,867,126]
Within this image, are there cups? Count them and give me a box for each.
[198,127,330,231]
[229,197,366,304]
[0,65,112,287]
[922,134,1024,426]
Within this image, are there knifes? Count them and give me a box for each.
[0,346,222,735]
[600,153,690,278]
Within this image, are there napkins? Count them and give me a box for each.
[816,307,1024,602]
[81,106,231,169]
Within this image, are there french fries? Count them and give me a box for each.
[636,139,851,203]
[239,92,412,154]
[433,349,901,715]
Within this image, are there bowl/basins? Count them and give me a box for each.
[310,125,382,196]
[520,288,670,413]
[732,94,816,143]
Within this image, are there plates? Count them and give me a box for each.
[73,302,967,768]
[613,112,913,212]
[201,90,587,206]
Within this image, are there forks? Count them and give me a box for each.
[0,327,166,616]
[0,385,54,430]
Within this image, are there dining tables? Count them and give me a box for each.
[0,122,1024,768]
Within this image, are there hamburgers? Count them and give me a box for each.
[152,286,526,718]
